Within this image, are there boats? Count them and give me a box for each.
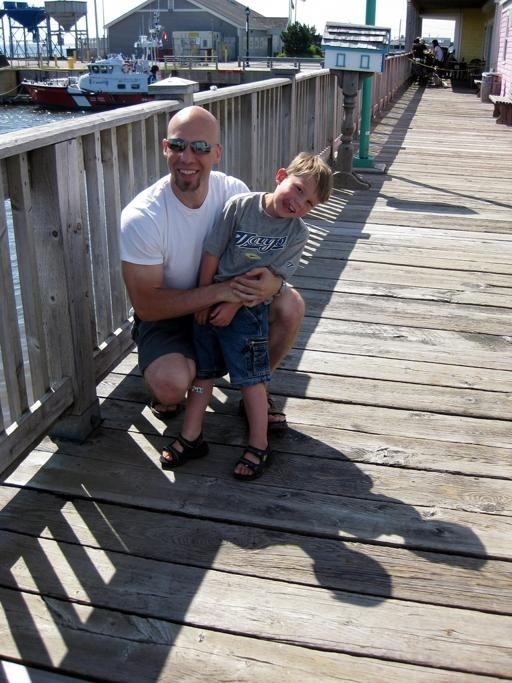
[0,52,22,104]
[22,58,158,109]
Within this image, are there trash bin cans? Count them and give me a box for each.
[480,72,501,103]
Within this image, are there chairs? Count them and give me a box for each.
[409,52,485,88]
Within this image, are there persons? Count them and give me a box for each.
[412,35,446,84]
[119,103,302,439]
[160,150,335,481]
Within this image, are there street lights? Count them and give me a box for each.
[243,7,251,66]
[294,0,307,30]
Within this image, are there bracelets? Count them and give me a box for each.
[273,273,286,298]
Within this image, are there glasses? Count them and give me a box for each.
[166,138,219,156]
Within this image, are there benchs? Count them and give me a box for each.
[487,94,512,124]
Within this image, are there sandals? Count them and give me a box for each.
[239,391,288,429]
[233,441,274,481]
[150,394,182,420]
[159,428,207,467]
